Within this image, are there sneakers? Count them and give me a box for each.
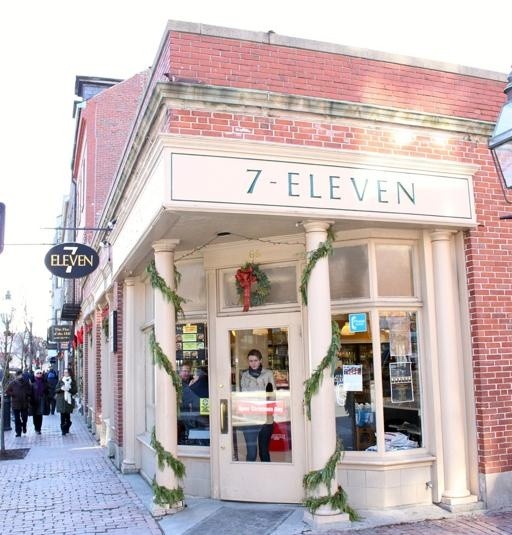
[61,422,72,434]
[15,426,26,437]
[36,429,42,434]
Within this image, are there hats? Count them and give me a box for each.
[34,370,43,376]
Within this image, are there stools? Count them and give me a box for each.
[356,425,376,450]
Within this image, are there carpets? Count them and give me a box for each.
[182,506,296,534]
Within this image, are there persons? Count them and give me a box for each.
[240,349,277,462]
[6,368,77,437]
[179,363,209,446]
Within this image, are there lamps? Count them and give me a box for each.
[99,240,107,248]
[107,218,116,230]
[486,64,512,221]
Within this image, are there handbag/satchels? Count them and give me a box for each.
[39,397,50,415]
